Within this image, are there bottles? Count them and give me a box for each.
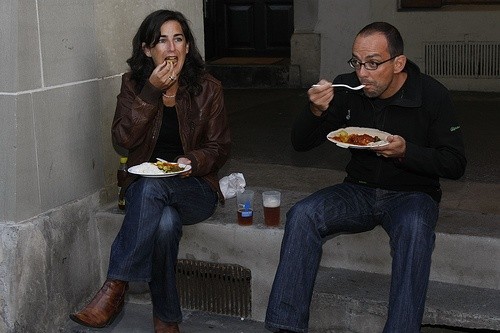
[117,158,131,210]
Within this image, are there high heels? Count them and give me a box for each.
[70,278,129,328]
[153,315,180,333]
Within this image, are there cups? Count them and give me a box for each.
[262,191,281,227]
[237,190,254,226]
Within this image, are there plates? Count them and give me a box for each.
[128,163,191,177]
[327,127,392,148]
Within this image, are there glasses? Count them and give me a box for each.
[348,55,398,70]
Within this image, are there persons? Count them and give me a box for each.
[69,8,234,332]
[265,20,468,333]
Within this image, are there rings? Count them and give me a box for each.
[377,152,380,156]
[169,75,174,80]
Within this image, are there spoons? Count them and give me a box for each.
[313,84,366,90]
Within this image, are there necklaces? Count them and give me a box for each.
[162,91,176,97]
